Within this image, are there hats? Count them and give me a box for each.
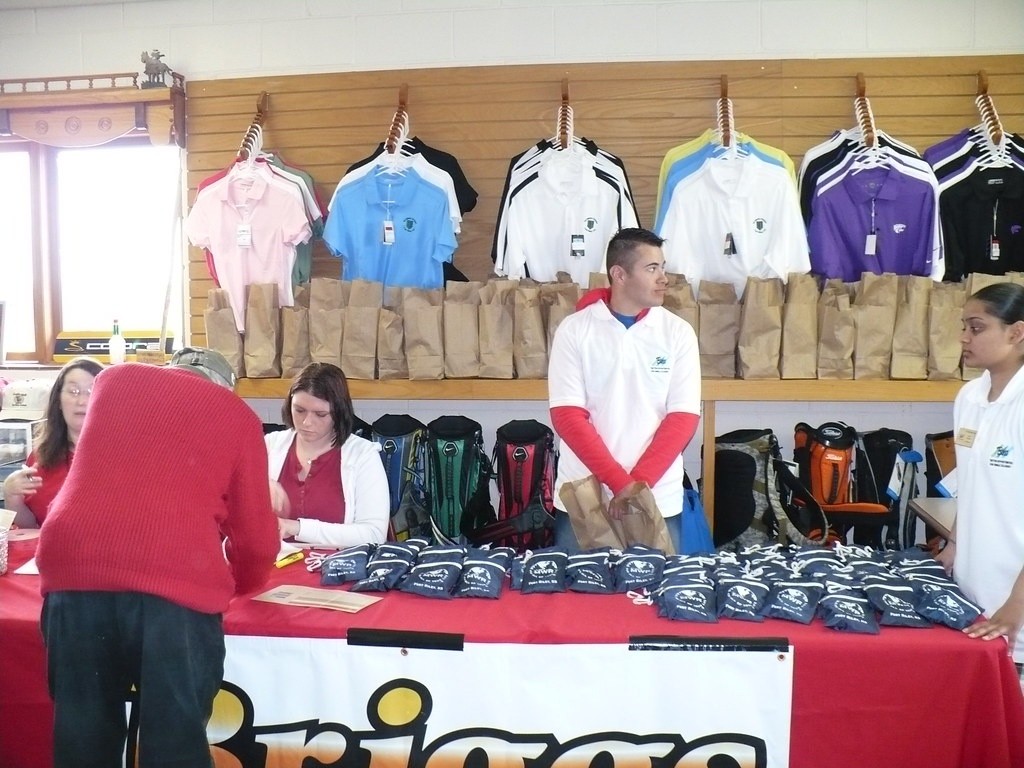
[170,347,237,387]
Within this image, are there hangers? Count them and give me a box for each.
[972,93,1015,169]
[380,110,416,205]
[844,96,893,176]
[227,124,275,208]
[547,105,587,193]
[711,98,750,182]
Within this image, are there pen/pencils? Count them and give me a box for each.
[21,464,33,483]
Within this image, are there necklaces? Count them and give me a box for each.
[298,435,324,465]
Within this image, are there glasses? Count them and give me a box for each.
[61,387,91,398]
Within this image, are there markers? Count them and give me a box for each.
[276,552,304,569]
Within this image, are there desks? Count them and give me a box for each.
[0,550,1024,768]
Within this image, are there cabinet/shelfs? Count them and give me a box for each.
[230,377,970,551]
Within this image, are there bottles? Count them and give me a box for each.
[109,319,126,364]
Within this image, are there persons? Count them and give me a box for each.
[934,283,1023,678]
[548,228,702,555]
[4,347,390,768]
